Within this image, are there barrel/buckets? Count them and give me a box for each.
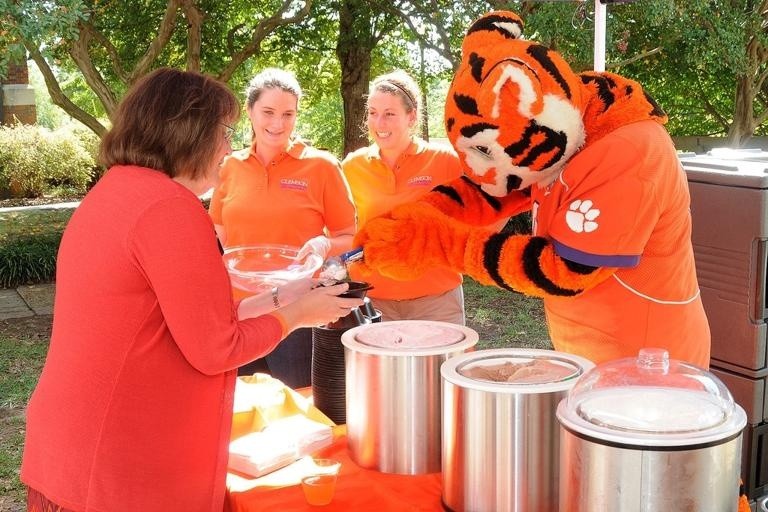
[341,318,479,475]
[441,346,592,512]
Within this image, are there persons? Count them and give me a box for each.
[20,66,366,512]
[208,67,359,391]
[340,69,469,329]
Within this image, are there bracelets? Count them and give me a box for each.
[270,286,282,311]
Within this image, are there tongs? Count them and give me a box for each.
[341,242,365,266]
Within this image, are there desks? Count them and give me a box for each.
[220,383,751,512]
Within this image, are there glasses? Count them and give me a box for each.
[217,122,236,142]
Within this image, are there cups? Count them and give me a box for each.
[296,458,343,506]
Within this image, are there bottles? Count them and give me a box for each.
[236,277,339,321]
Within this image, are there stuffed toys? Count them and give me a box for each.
[350,10,752,511]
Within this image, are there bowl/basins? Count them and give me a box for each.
[220,242,323,296]
[309,297,382,427]
[317,277,374,306]
